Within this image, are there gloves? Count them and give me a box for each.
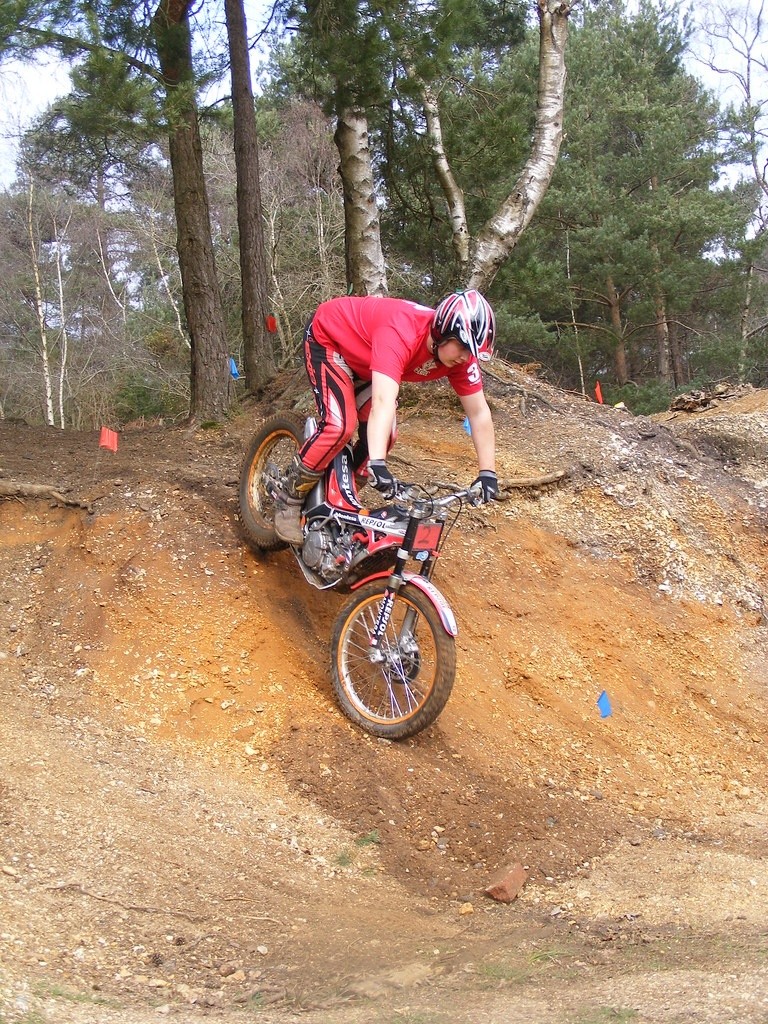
[470,470,499,504]
[366,459,399,500]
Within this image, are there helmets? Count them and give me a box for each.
[430,289,497,362]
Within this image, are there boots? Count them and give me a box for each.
[271,455,325,544]
[353,471,367,496]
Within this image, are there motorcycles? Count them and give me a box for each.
[236,408,483,741]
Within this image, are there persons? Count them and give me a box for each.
[271,288,500,543]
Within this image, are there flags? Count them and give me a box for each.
[597,691,611,717]
[595,381,603,404]
[464,417,471,435]
[230,358,240,378]
[99,427,118,451]
